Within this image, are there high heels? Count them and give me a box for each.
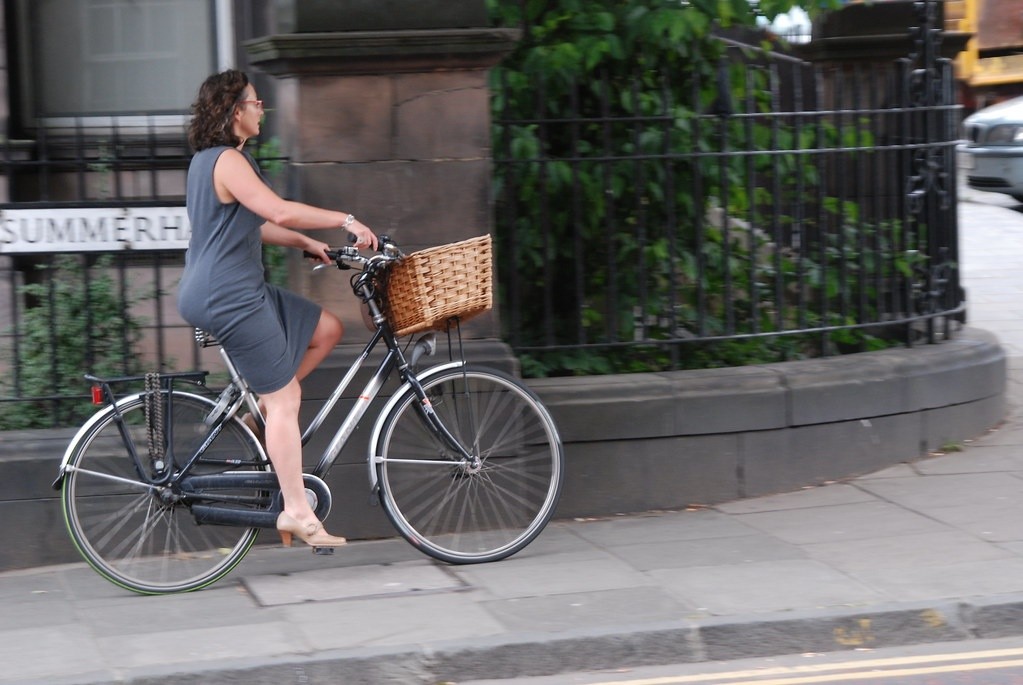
[241,413,262,437]
[276,510,347,549]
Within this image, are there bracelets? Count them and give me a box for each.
[339,213,355,233]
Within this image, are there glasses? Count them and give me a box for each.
[235,99,264,109]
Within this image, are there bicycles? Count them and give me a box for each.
[51,231,565,595]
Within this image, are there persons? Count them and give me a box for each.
[173,65,386,553]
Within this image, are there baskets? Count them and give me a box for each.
[370,232,492,341]
